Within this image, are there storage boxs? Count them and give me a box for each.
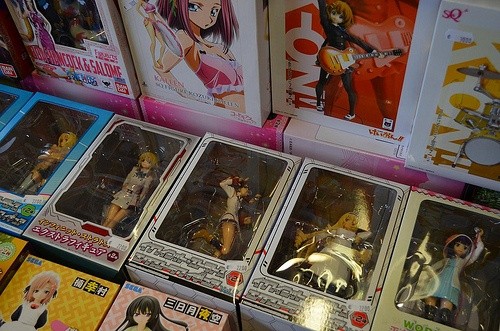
[0,92,115,235]
[21,114,203,284]
[238,156,411,330]
[0,254,122,331]
[369,183,500,331]
[139,94,291,152]
[96,280,232,331]
[0,232,29,297]
[5,0,140,100]
[284,117,465,198]
[118,0,271,128]
[125,130,302,331]
[268,0,443,144]
[405,1,500,192]
[31,68,143,121]
[1,1,35,132]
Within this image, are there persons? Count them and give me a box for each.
[187,174,250,259]
[102,152,160,231]
[422,227,485,325]
[16,131,77,196]
[293,212,375,296]
